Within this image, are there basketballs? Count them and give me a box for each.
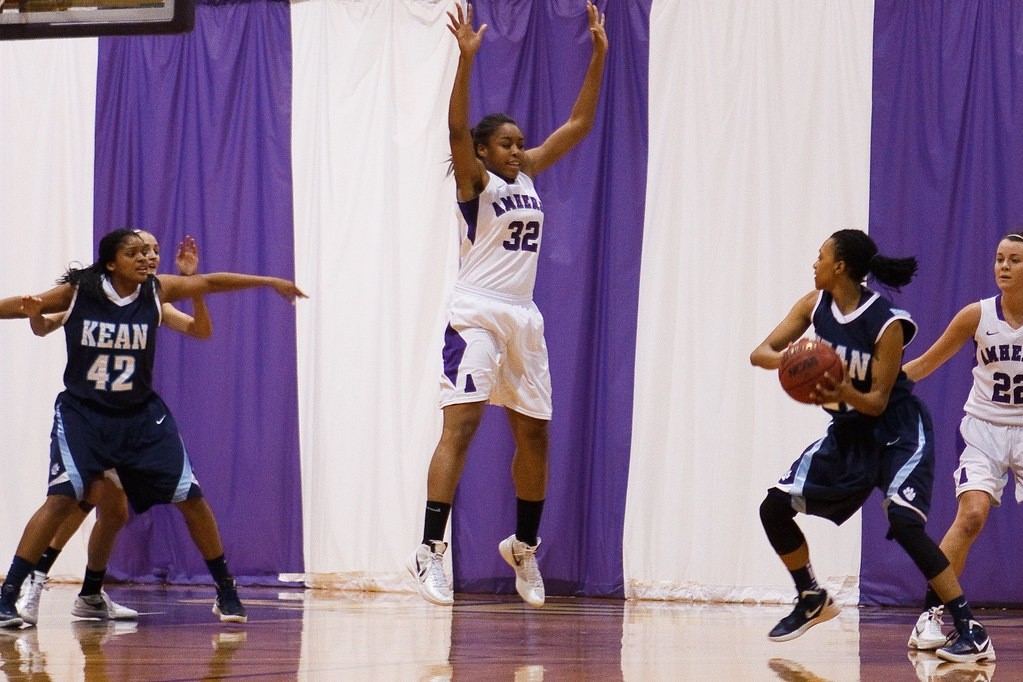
[777,339,843,406]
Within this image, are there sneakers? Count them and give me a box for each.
[0,584,24,627]
[498,532,545,607]
[16,570,49,623]
[71,586,138,617]
[212,578,248,623]
[407,540,454,606]
[936,615,997,662]
[769,587,841,642]
[907,603,953,648]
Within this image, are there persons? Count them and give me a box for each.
[0,227,310,630]
[750,228,997,665]
[404,0,609,605]
[902,232,1023,651]
